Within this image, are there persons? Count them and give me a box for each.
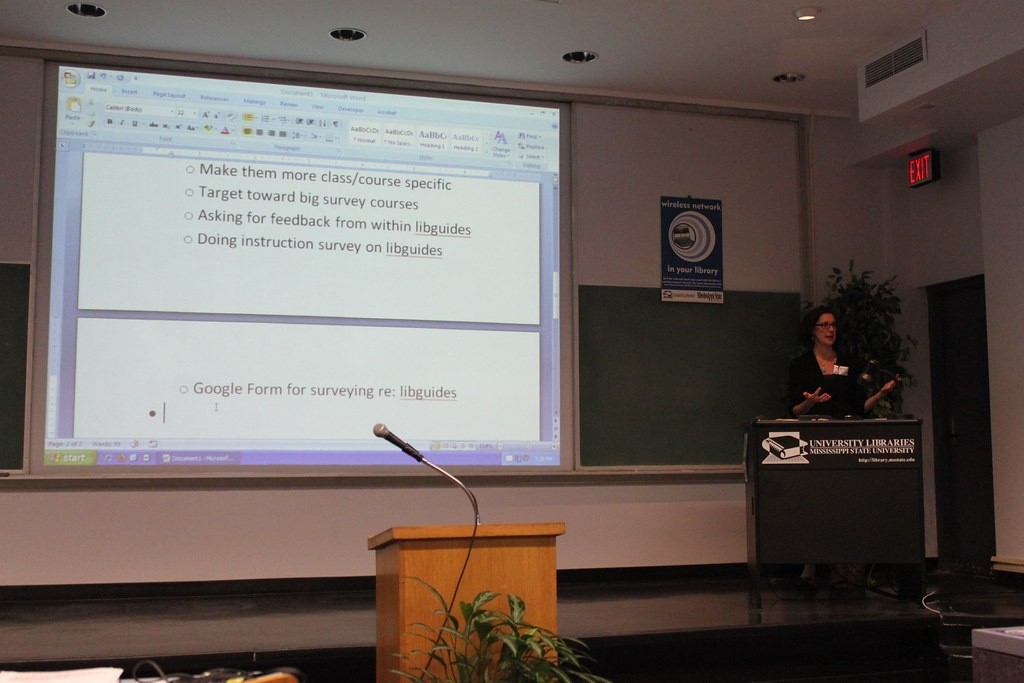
[787,305,903,416]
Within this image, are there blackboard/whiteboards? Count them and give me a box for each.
[573,281,806,472]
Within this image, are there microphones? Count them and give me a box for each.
[373,424,423,462]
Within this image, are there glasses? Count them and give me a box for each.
[815,322,837,329]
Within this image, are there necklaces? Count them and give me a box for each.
[815,354,831,374]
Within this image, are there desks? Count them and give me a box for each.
[742,418,927,608]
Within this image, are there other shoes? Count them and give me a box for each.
[837,567,864,587]
[797,563,816,589]
